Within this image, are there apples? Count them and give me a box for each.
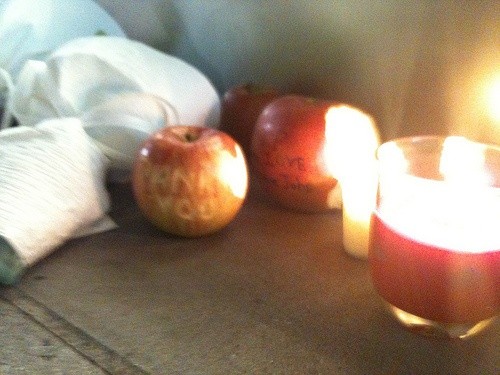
[132,124,250,240]
[222,80,277,137]
[251,95,363,212]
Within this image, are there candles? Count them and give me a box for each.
[366,133,500,338]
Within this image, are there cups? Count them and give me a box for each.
[369,135,499,323]
